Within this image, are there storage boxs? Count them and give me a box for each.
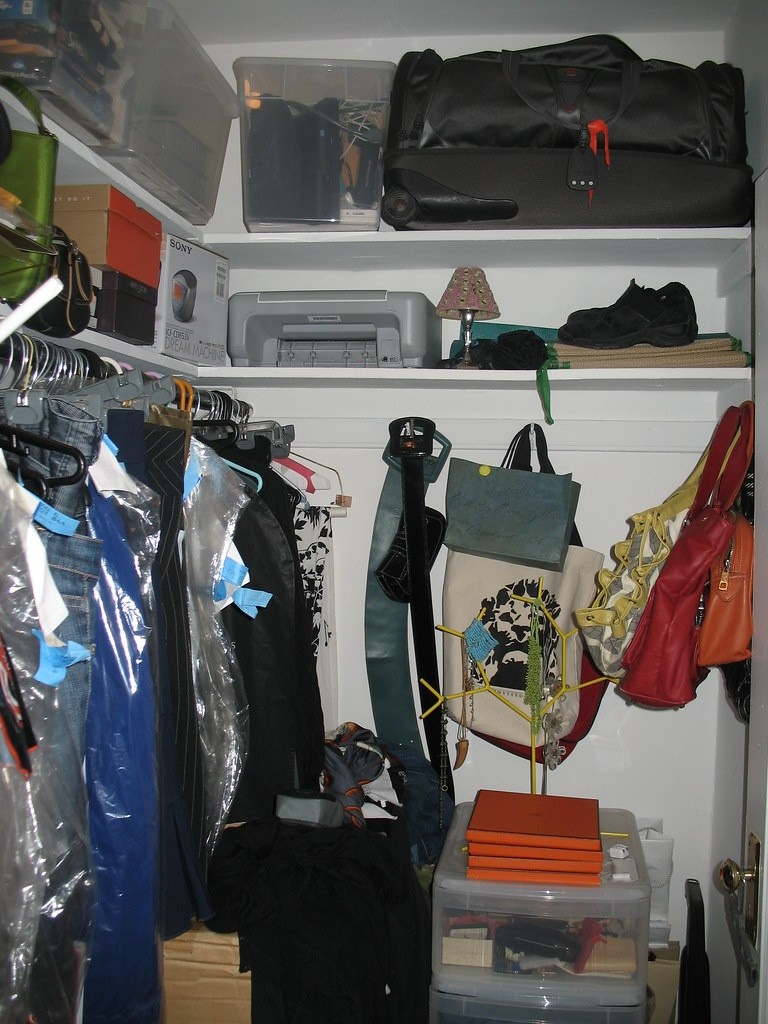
[159,917,252,1024]
[0,0,243,226]
[87,264,103,327]
[96,272,159,345]
[53,182,163,285]
[152,233,230,367]
[232,56,397,233]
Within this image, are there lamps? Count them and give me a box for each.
[435,267,502,369]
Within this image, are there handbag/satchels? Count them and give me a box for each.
[373,505,446,603]
[439,423,606,770]
[8,225,93,340]
[574,400,755,726]
[0,75,59,303]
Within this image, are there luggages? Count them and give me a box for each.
[237,723,454,1024]
[378,32,754,227]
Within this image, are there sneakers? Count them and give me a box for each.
[556,277,699,349]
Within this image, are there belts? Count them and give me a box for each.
[365,425,452,768]
[389,416,458,804]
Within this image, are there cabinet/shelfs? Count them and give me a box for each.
[429,801,650,1024]
[0,85,755,395]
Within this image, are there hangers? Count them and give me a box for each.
[1,334,331,499]
